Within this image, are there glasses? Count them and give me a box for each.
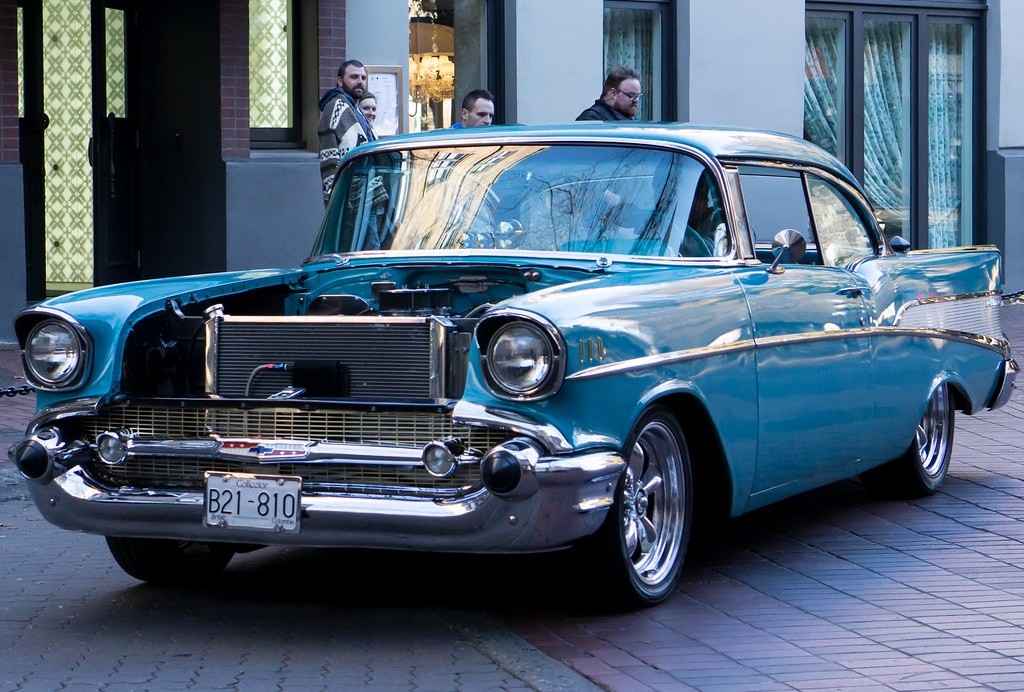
[616,87,644,101]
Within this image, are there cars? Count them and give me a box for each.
[8,121,1019,610]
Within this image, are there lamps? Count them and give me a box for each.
[409,16,457,105]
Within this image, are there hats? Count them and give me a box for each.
[652,153,710,202]
[359,91,375,103]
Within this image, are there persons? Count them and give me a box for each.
[317,59,390,251]
[559,152,723,258]
[358,91,377,125]
[447,89,495,129]
[575,67,641,120]
